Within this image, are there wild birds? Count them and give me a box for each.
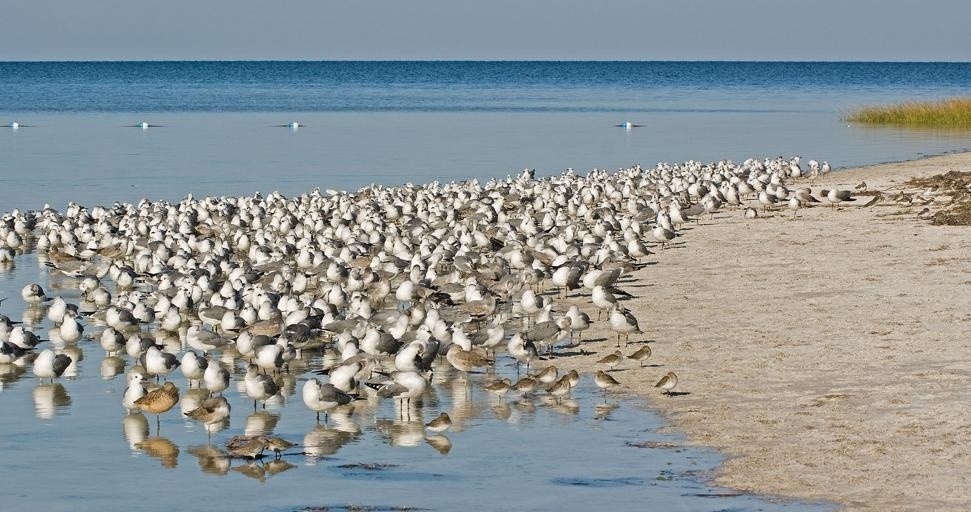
[864,191,882,212]
[826,189,851,207]
[855,181,867,192]
[893,177,971,218]
[0,155,830,461]
